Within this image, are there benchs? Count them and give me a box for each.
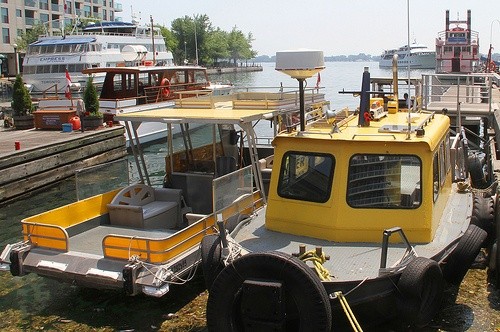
[29,103,78,131]
[106,183,183,229]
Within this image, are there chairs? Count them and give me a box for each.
[216,155,256,207]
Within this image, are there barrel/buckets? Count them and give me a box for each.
[69,116,80,129]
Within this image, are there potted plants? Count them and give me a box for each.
[82,76,105,130]
[10,73,33,130]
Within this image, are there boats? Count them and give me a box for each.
[0,82,330,299]
[21,16,174,99]
[82,16,236,150]
[366,10,500,114]
[378,38,437,69]
[202,0,474,332]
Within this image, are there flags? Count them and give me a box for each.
[65,70,72,99]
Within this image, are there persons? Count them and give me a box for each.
[404,93,412,108]
[378,90,389,103]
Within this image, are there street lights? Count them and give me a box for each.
[491,19,500,43]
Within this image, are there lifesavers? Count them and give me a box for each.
[206,249,333,332]
[391,256,444,322]
[160,77,171,97]
[444,223,488,288]
[488,62,496,71]
[467,157,494,248]
